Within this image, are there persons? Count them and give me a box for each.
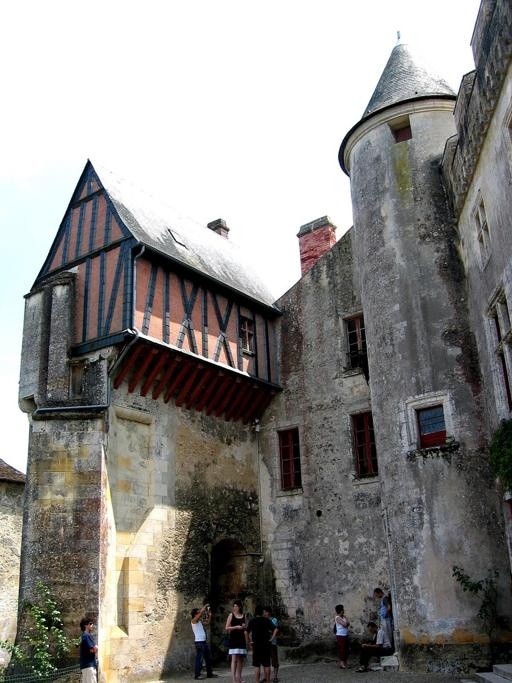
[244,607,278,683]
[225,600,248,683]
[355,622,394,672]
[335,605,351,669]
[80,617,98,683]
[260,605,279,683]
[374,588,393,644]
[191,604,218,680]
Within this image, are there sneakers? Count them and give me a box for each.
[273,678,278,683]
[340,665,366,672]
[198,676,205,680]
[260,679,266,683]
[207,673,218,678]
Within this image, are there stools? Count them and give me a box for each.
[380,656,400,672]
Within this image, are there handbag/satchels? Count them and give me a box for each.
[333,624,337,634]
